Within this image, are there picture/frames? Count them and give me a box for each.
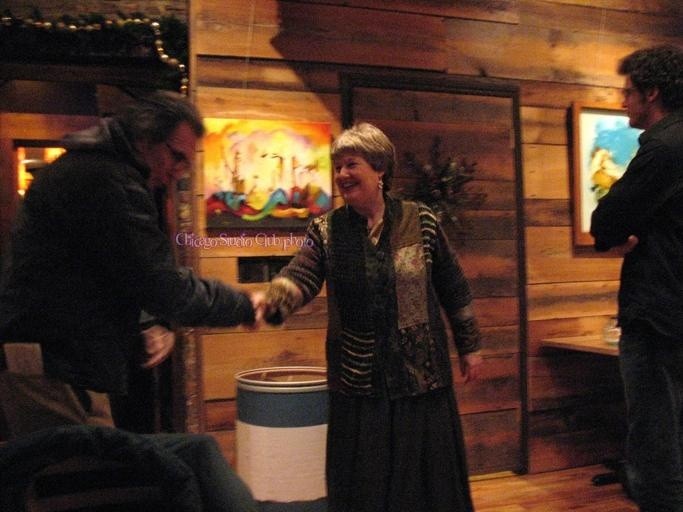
[573,99,646,245]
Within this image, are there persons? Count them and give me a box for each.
[588,41,682,512]
[251,120,484,512]
[0,87,272,435]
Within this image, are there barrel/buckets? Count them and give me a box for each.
[236,367,330,512]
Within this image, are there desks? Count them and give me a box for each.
[543,334,626,485]
